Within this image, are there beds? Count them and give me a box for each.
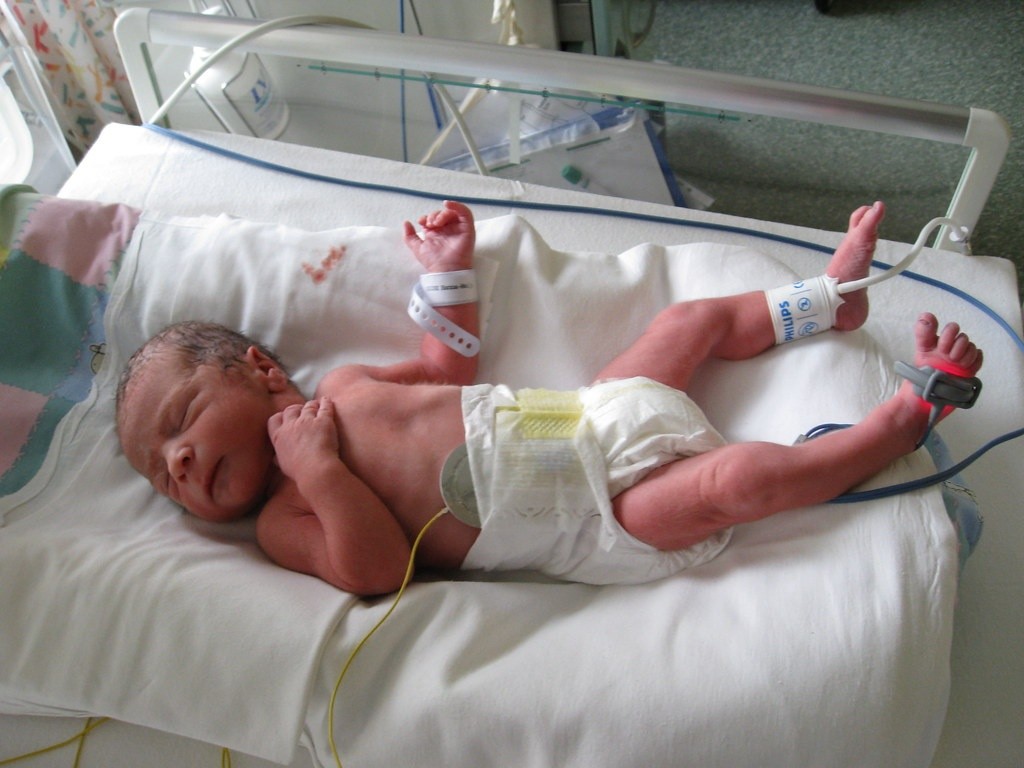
[0,7,1024,768]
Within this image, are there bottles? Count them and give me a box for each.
[190,6,290,138]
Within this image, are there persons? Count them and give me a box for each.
[116,199,985,597]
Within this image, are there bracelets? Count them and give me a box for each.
[407,266,481,359]
